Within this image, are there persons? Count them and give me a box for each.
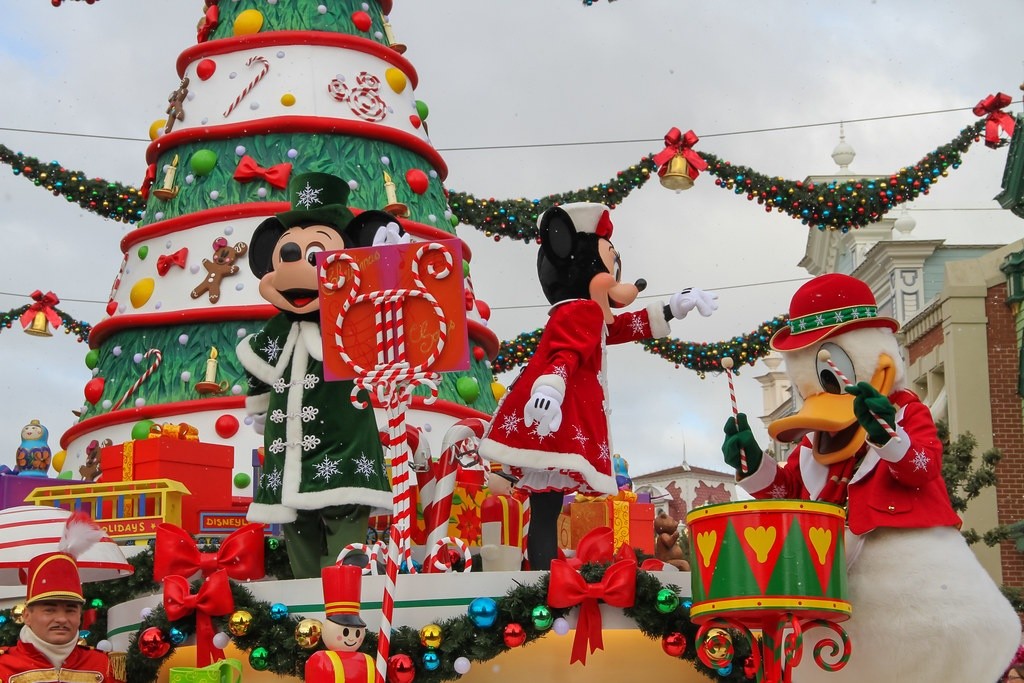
[1007,664,1024,683]
[304,565,379,683]
[0,551,112,683]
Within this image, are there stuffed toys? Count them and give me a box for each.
[479,201,721,571]
[653,508,689,571]
[720,272,1021,683]
[231,171,395,580]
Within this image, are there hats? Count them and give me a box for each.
[26,512,106,606]
